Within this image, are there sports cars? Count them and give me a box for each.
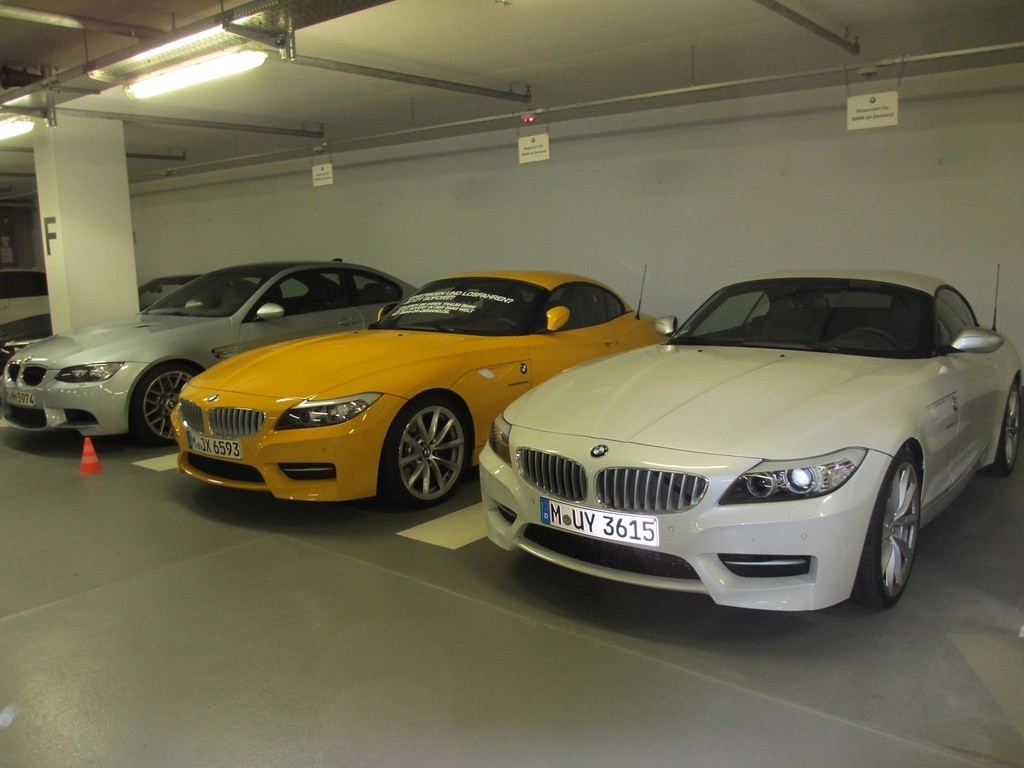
[473,267,1024,613]
[0,256,420,447]
[168,265,672,511]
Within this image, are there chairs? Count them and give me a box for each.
[484,288,605,328]
[757,295,921,342]
[307,280,381,308]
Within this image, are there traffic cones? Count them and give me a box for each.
[77,437,107,478]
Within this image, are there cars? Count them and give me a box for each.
[0,264,258,380]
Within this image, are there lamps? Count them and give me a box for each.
[121,42,273,104]
[0,114,38,140]
